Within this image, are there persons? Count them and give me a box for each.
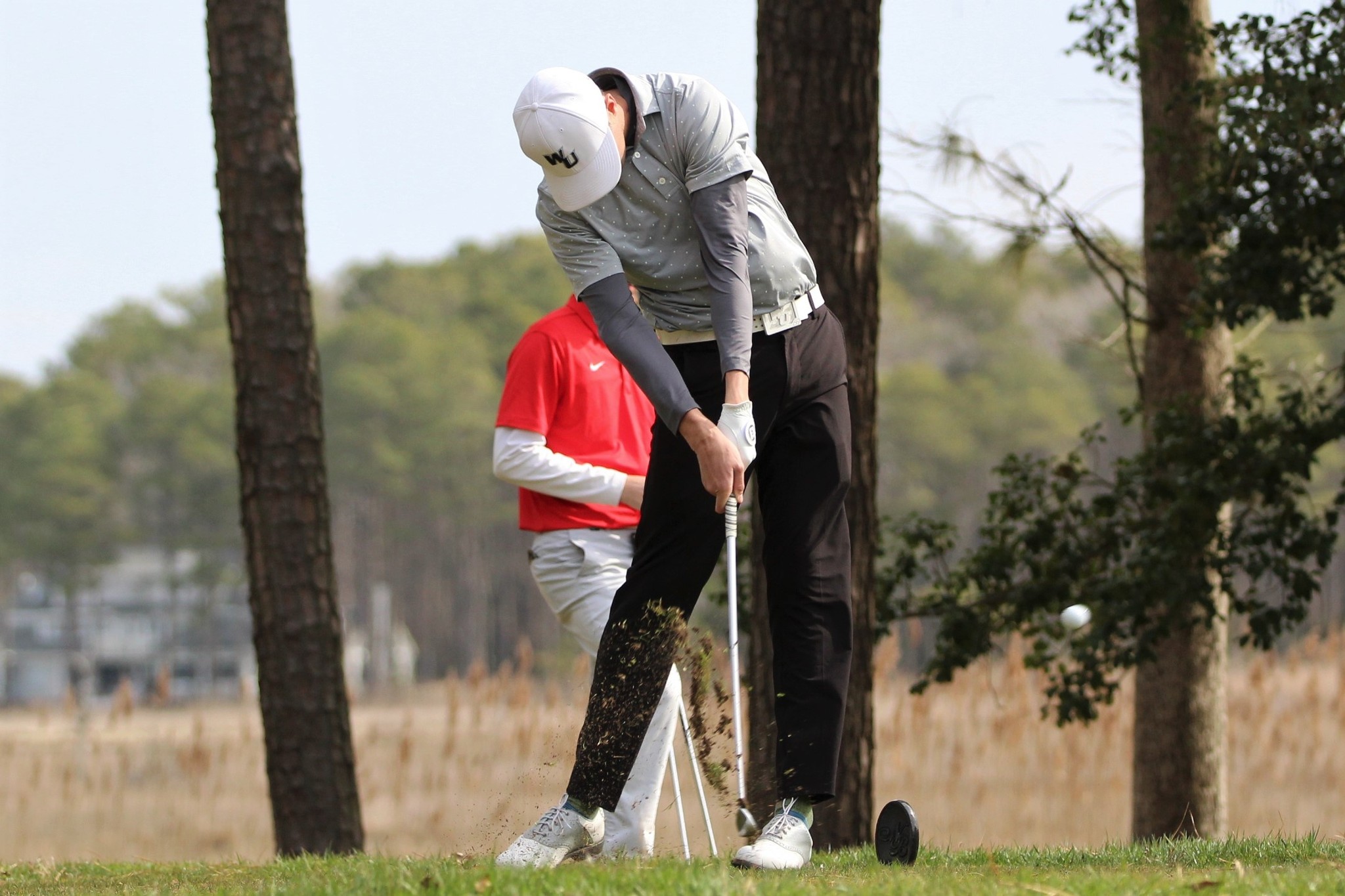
[513,66,853,872]
[493,281,682,861]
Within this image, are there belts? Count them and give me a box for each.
[646,284,825,345]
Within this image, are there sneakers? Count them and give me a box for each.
[731,798,813,872]
[495,793,605,869]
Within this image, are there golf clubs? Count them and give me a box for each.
[723,493,759,840]
[668,742,691,864]
[680,700,719,860]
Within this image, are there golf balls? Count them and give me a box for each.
[1059,603,1093,630]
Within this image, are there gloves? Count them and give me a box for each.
[716,402,756,471]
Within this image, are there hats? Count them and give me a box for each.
[513,67,621,213]
